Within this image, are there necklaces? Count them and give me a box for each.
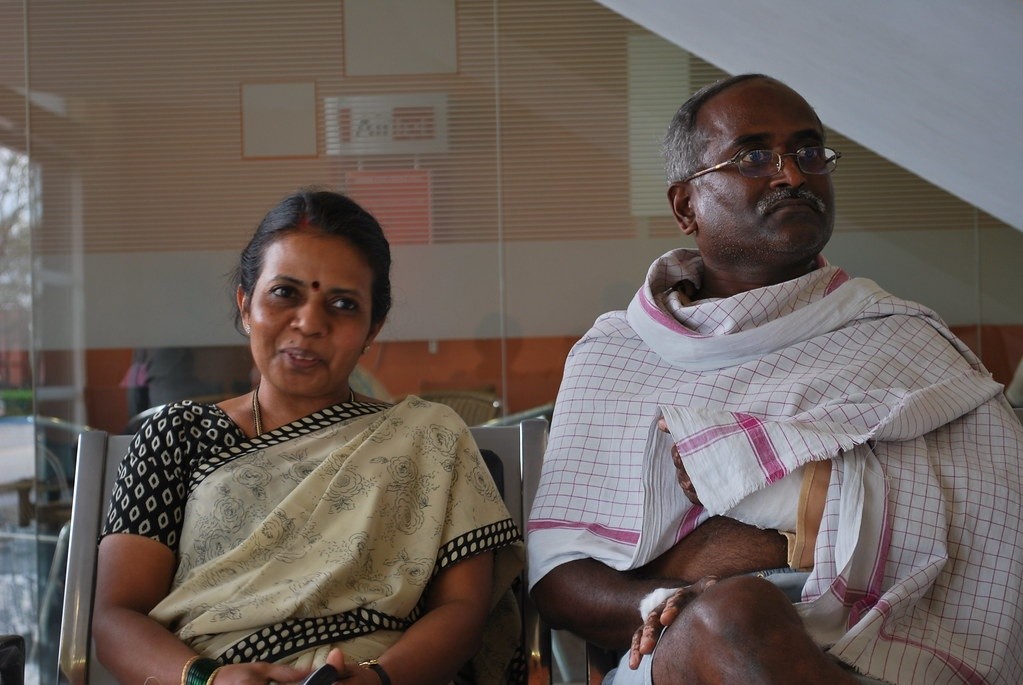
[252,385,354,436]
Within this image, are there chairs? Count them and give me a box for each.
[62,413,552,685]
[395,390,506,424]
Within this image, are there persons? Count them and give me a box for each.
[527,72,1023,685]
[85,190,526,684]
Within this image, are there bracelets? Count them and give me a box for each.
[180,655,224,685]
[359,659,392,685]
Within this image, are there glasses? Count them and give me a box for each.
[682,143,841,183]
